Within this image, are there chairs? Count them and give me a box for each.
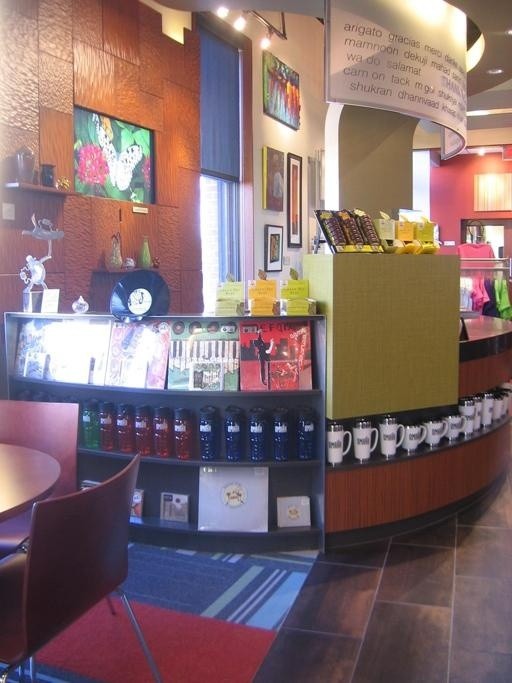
[0,399,83,553]
[0,449,161,683]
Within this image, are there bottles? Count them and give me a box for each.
[102,232,122,273]
[80,398,318,461]
[138,235,152,268]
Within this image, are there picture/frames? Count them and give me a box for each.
[287,152,303,247]
[264,224,283,272]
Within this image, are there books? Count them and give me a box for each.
[13,319,312,392]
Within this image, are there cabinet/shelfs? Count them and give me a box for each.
[3,308,324,554]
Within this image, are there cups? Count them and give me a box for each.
[457,382,512,436]
[326,411,468,463]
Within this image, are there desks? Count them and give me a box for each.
[1,440,63,524]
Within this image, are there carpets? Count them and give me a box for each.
[2,544,321,682]
